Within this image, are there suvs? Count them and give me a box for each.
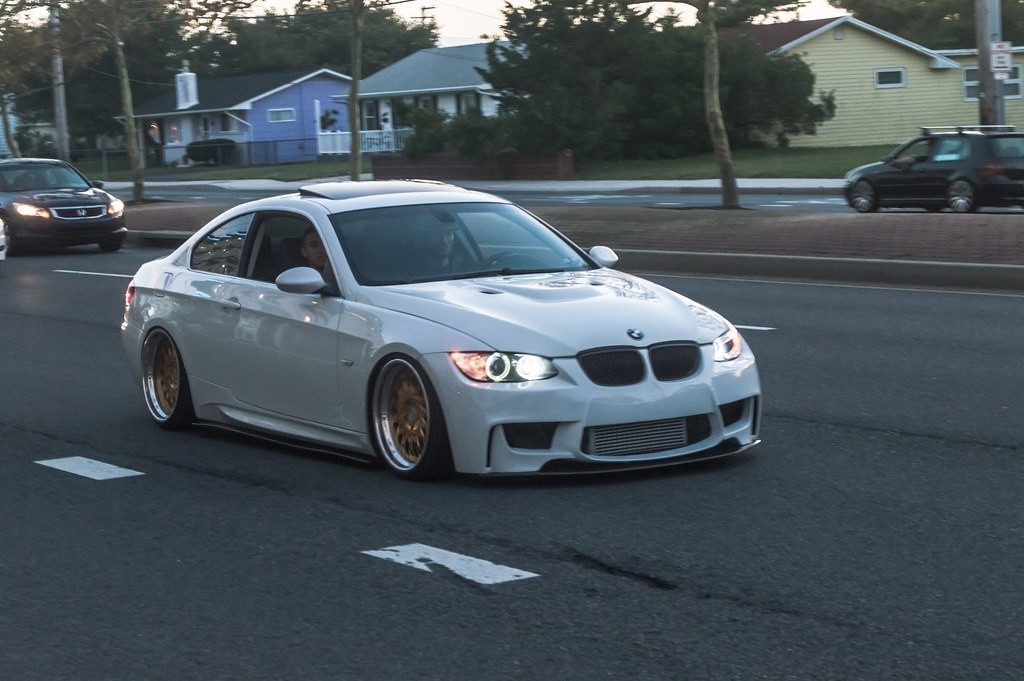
[843,126,1024,213]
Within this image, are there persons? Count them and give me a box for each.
[409,211,477,277]
[298,226,329,274]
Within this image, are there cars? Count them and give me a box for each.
[119,178,763,480]
[0,157,128,255]
